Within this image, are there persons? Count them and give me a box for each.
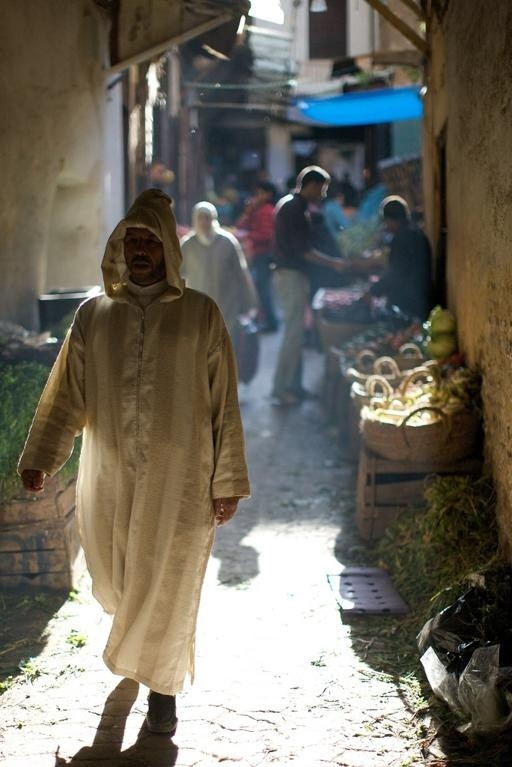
[17,185,251,738]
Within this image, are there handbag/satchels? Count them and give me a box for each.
[235,315,260,384]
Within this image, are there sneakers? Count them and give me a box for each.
[144,687,179,733]
[274,386,319,406]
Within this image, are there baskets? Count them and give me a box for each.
[345,342,479,467]
